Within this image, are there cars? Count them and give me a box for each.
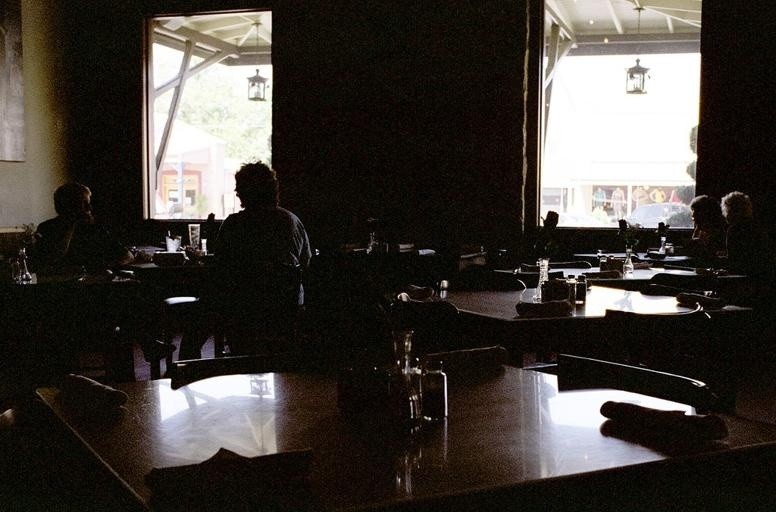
[628,201,693,229]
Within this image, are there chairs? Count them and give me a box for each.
[0,217,776,512]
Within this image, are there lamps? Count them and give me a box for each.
[247,15,266,101]
[626,7,645,94]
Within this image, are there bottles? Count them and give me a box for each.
[200,232,207,253]
[410,357,449,421]
[599,255,614,263]
[567,274,587,302]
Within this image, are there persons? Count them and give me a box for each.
[31,182,101,278]
[177,165,310,362]
[650,186,666,204]
[611,187,626,220]
[632,187,649,208]
[594,187,607,210]
[691,192,758,273]
